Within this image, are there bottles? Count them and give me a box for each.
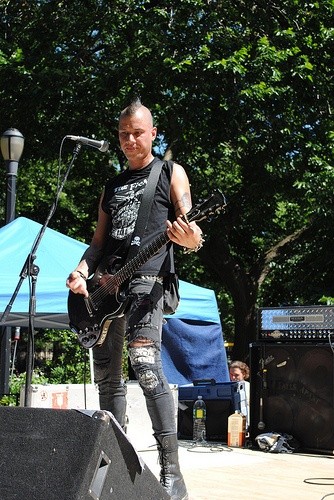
[193,396,207,441]
[227,410,246,448]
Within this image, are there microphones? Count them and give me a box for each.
[257,397,266,431]
[65,134,110,153]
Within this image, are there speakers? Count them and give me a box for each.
[248,342,334,457]
[0,404,172,500]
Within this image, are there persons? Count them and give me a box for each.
[229,361,250,426]
[66,98,202,500]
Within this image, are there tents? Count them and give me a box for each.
[0,216,228,385]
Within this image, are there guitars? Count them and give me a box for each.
[67,187,228,349]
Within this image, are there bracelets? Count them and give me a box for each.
[77,271,87,280]
[183,238,205,254]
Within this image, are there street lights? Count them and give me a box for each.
[0,126,24,405]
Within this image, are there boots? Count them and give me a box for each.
[153,433,189,500]
